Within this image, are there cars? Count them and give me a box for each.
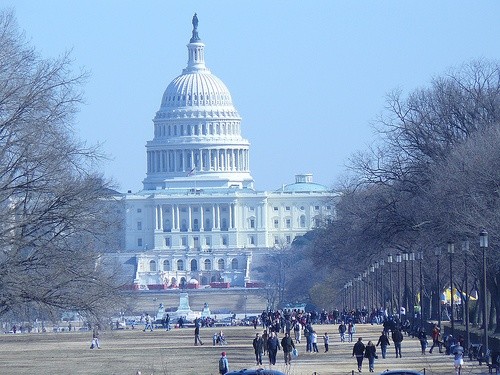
[152,314,262,328]
[223,367,287,375]
[78,327,89,331]
[108,318,136,330]
[379,369,427,375]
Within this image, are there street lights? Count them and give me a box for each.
[434,244,442,328]
[446,238,454,341]
[370,264,375,310]
[417,249,424,326]
[459,237,471,352]
[387,253,393,314]
[395,252,402,321]
[336,265,371,313]
[374,260,380,310]
[379,257,385,311]
[409,249,416,323]
[479,229,489,349]
[402,249,409,315]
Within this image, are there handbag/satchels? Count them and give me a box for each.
[293,349,299,357]
[422,339,427,348]
[222,368,227,374]
[375,354,378,359]
[305,331,309,337]
[438,335,443,342]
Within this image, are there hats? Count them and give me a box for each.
[222,352,226,356]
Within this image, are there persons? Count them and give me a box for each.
[218,351,229,375]
[418,327,428,355]
[338,321,347,342]
[193,322,204,346]
[319,307,385,326]
[376,332,390,359]
[165,314,171,331]
[352,337,365,372]
[383,315,420,338]
[178,318,184,328]
[267,332,281,365]
[89,326,101,349]
[11,325,31,333]
[281,332,295,365]
[194,317,215,328]
[68,324,71,331]
[323,333,329,353]
[393,327,404,358]
[212,330,227,346]
[453,342,464,375]
[252,309,321,356]
[348,321,355,342]
[143,314,153,332]
[253,333,264,365]
[429,325,444,354]
[365,340,376,373]
